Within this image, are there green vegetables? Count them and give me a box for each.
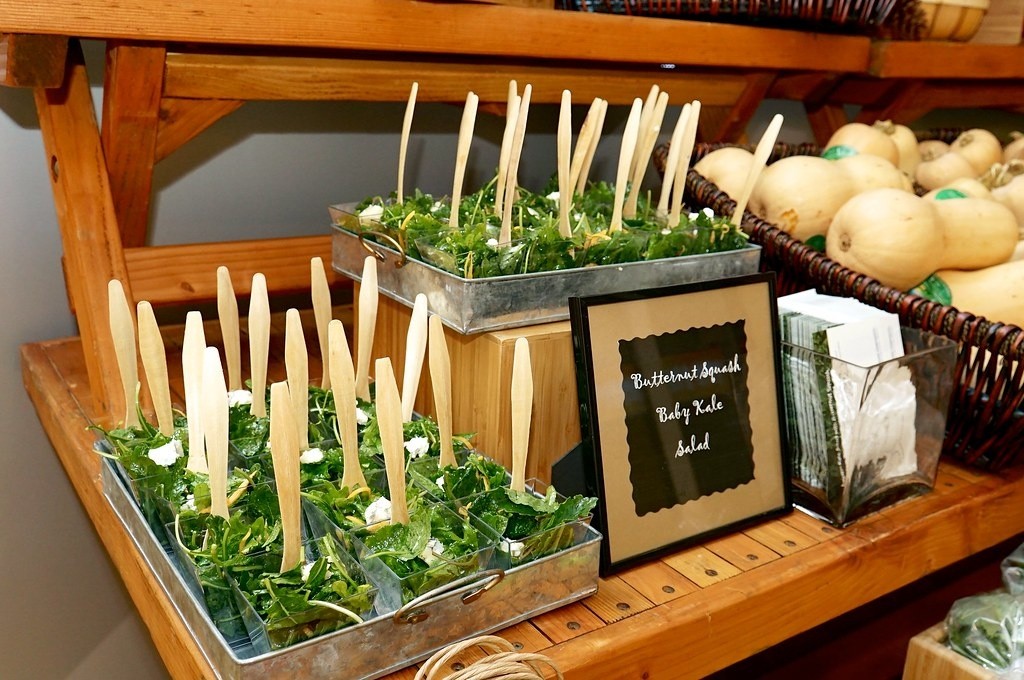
[83,377,599,649]
[329,163,749,281]
[942,588,1024,672]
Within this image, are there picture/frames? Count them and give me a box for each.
[574,270,793,585]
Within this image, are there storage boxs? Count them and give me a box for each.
[96,376,604,680]
[773,326,960,525]
[331,183,761,336]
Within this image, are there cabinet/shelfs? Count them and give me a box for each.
[0,0,1024,680]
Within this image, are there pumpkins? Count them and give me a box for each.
[689,119,1024,392]
